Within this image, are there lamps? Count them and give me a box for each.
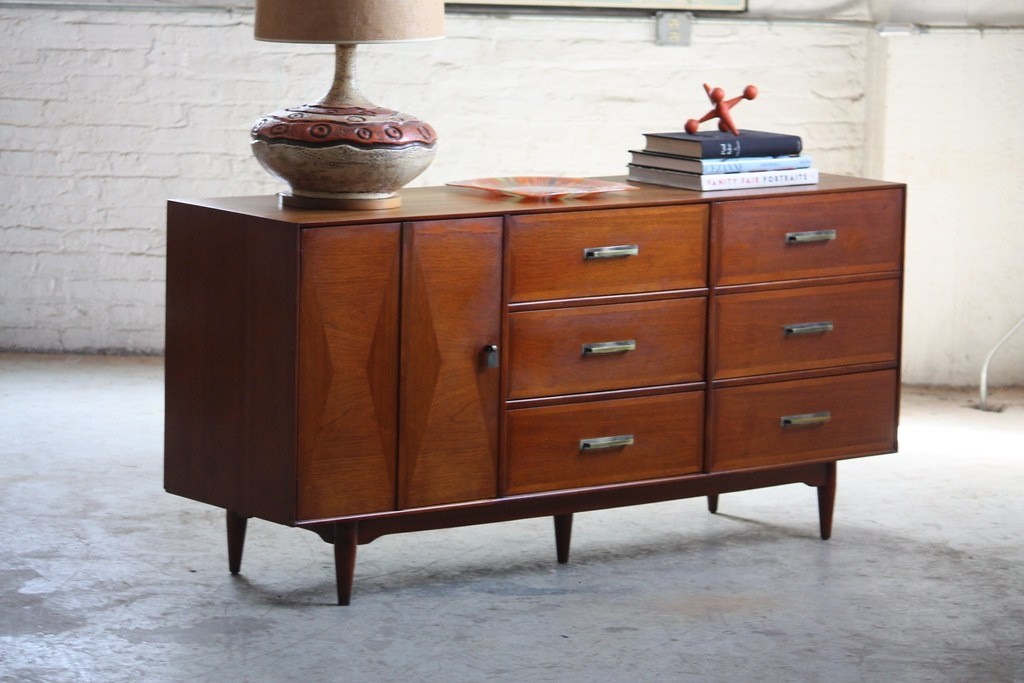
[251,0,448,209]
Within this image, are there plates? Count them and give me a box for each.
[446,176,640,202]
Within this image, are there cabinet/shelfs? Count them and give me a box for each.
[160,173,911,608]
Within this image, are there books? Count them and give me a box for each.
[642,128,802,159]
[626,149,820,192]
